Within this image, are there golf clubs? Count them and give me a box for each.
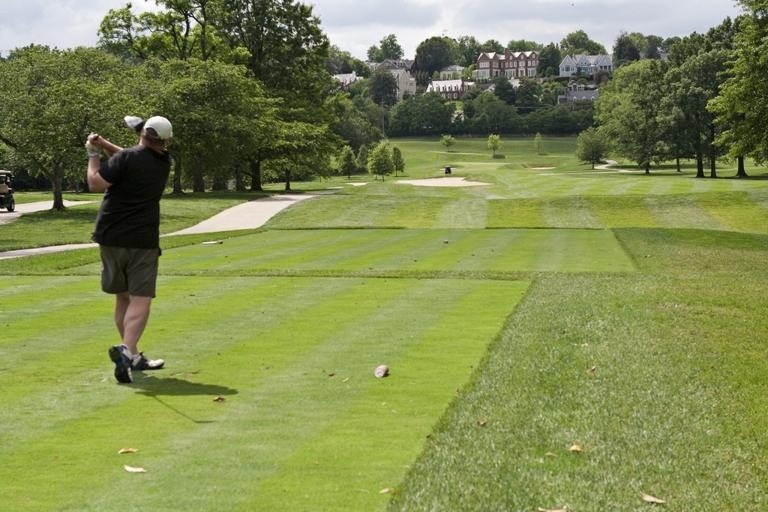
[93,114,144,142]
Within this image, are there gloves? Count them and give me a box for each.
[85,134,104,158]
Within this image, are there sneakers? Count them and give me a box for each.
[130,349,164,370]
[109,344,135,383]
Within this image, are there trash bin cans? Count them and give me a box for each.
[445,167,450,173]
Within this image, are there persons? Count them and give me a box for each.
[84,111,175,384]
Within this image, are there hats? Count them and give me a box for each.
[143,116,174,140]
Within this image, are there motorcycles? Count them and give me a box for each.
[0,170,15,211]
